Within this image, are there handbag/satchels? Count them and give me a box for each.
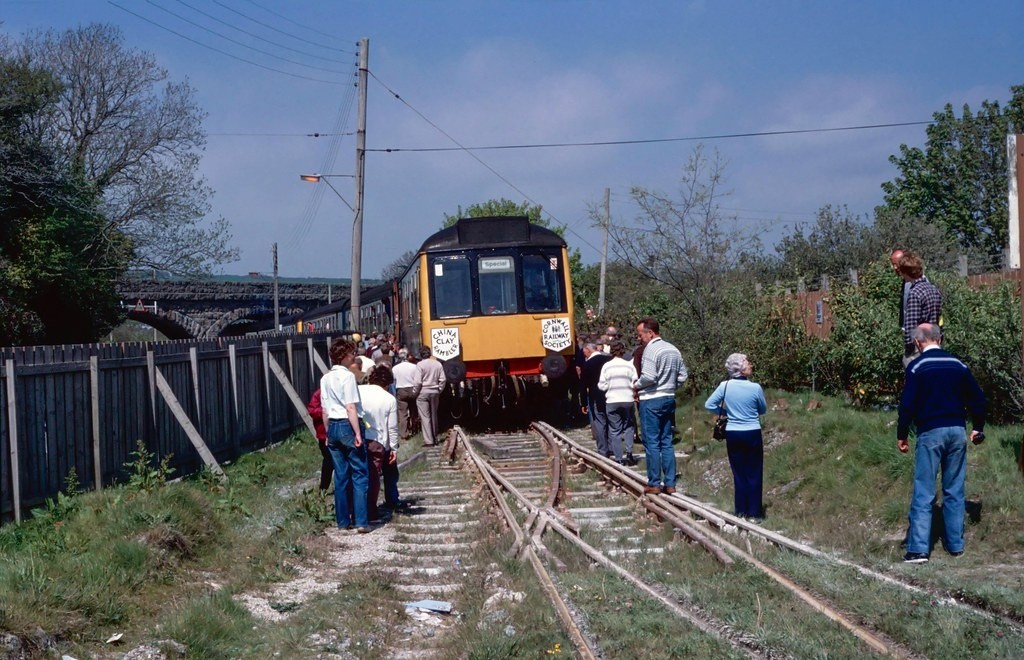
[713,418,728,442]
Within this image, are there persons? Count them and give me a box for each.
[632,315,688,494]
[307,331,446,497]
[320,338,407,533]
[569,308,645,466]
[890,247,944,368]
[896,323,988,563]
[705,352,767,524]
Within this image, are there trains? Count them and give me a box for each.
[218,215,576,425]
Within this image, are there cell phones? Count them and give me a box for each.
[972,432,986,445]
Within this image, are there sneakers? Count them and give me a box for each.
[644,485,662,494]
[903,552,930,563]
[943,536,965,561]
[337,526,371,535]
[736,516,763,524]
[663,484,675,493]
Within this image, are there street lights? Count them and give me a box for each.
[299,172,365,337]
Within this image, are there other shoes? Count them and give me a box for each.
[618,458,639,463]
[370,519,387,525]
[390,503,412,510]
[422,444,436,448]
